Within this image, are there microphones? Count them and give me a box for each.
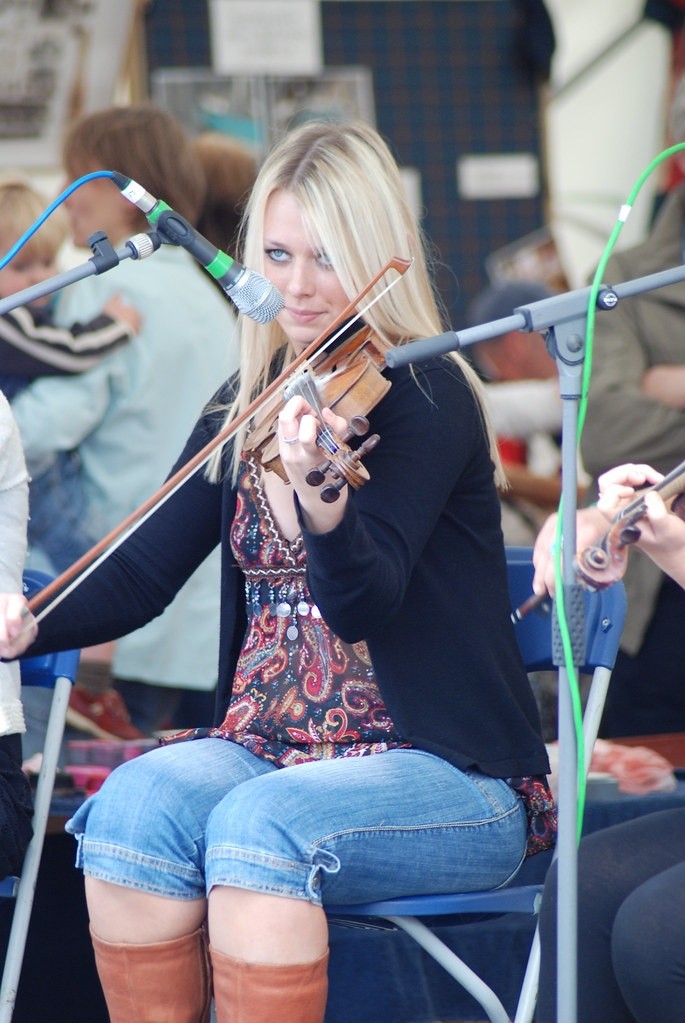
[110,171,284,325]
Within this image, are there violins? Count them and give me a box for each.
[571,461,685,587]
[242,324,382,503]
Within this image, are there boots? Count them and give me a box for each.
[89,910,211,1023]
[209,944,330,1023]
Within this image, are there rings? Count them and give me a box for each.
[282,432,300,449]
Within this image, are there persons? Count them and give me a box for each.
[536,73,685,1022]
[0,104,261,1023]
[0,116,558,1023]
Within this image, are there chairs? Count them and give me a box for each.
[326,546,624,1023]
[0,572,77,1023]
